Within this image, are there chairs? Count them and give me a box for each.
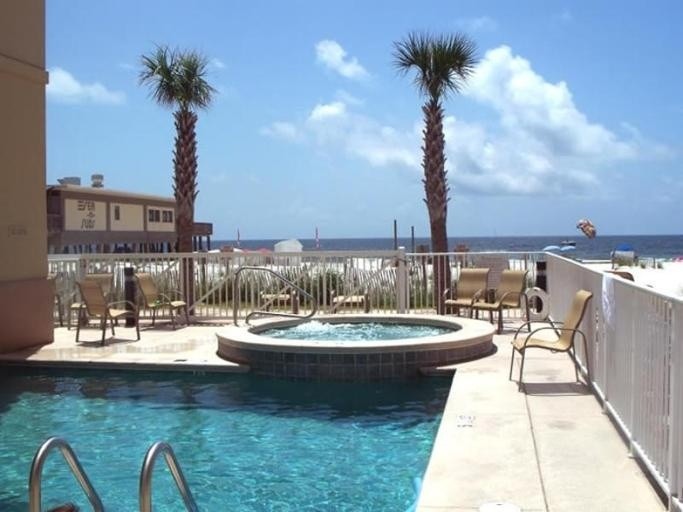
[260,277,299,315]
[508,288,594,393]
[48,272,189,346]
[331,271,370,314]
[442,267,490,318]
[471,268,531,334]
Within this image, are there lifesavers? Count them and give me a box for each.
[520,286,550,322]
